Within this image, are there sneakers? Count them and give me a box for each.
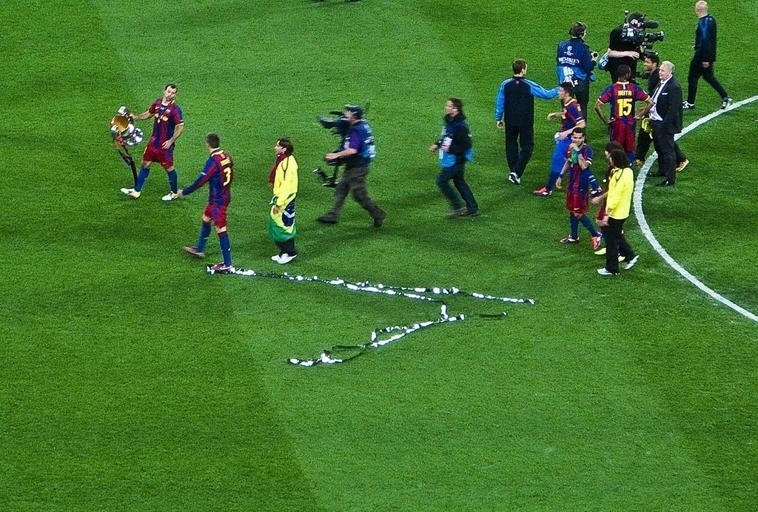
[121,188,141,198]
[594,247,606,255]
[446,207,467,219]
[676,158,690,172]
[272,255,280,261]
[559,234,580,244]
[647,172,666,177]
[207,263,235,274]
[532,187,553,198]
[596,268,620,275]
[459,209,479,216]
[630,159,646,167]
[318,212,339,224]
[720,97,733,110]
[277,253,298,264]
[623,254,640,269]
[591,232,602,250]
[161,191,178,201]
[507,172,521,185]
[184,246,205,258]
[618,256,625,262]
[682,100,695,109]
[587,186,604,200]
[656,179,675,187]
[373,210,387,228]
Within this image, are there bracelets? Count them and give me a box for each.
[576,151,581,154]
[559,174,564,178]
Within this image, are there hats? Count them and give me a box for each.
[345,105,363,116]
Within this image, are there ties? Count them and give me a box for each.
[653,83,662,101]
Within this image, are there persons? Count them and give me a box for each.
[268,138,298,266]
[556,22,598,144]
[428,97,479,221]
[314,105,388,230]
[608,11,646,83]
[120,84,181,203]
[595,64,653,167]
[495,58,572,185]
[634,53,690,173]
[534,83,603,196]
[645,61,683,187]
[590,141,640,275]
[177,134,234,272]
[681,1,732,110]
